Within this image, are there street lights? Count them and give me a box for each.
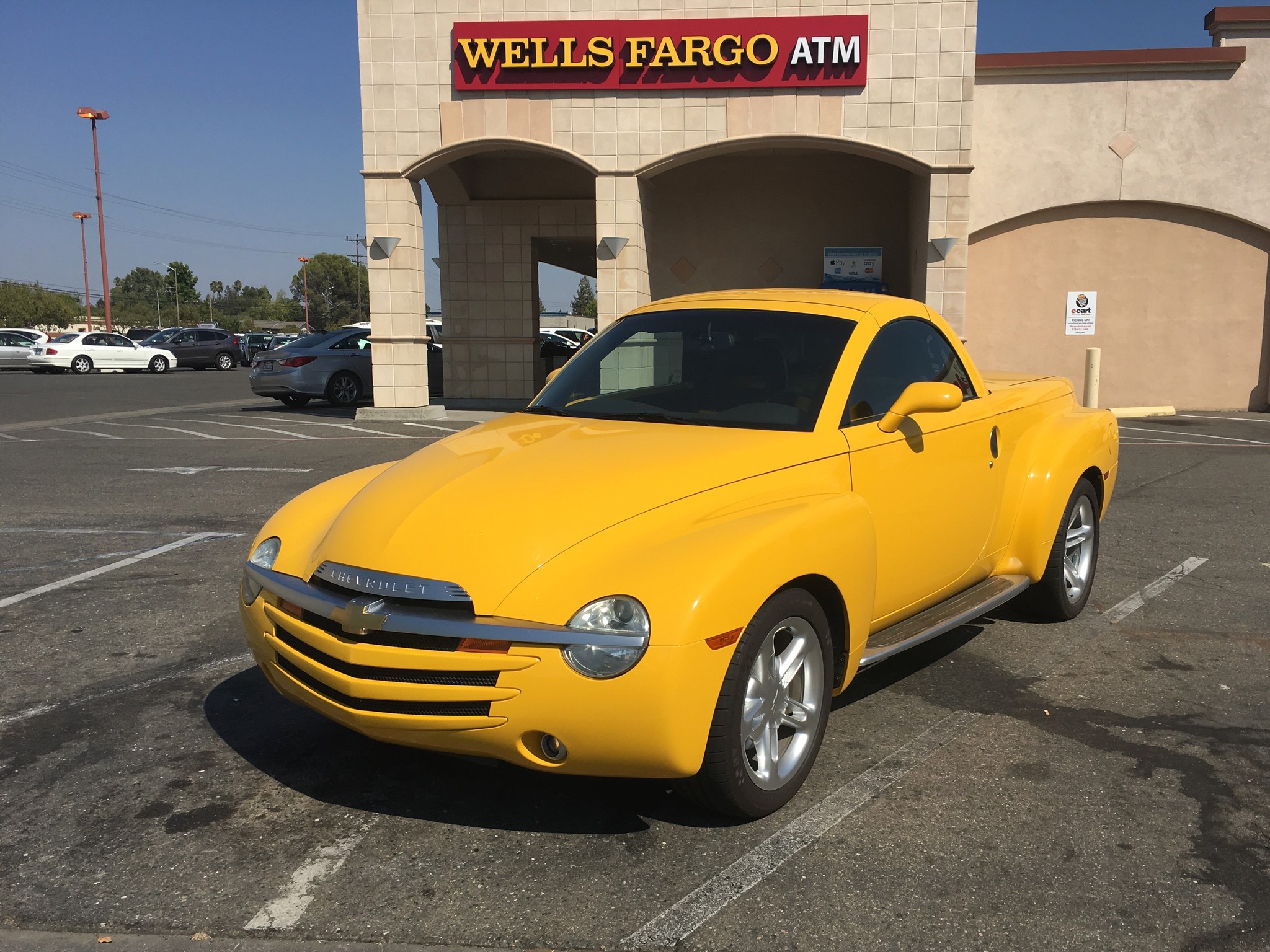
[71,210,93,332]
[77,106,115,334]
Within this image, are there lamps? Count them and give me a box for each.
[930,237,959,260]
[602,236,630,259]
[373,236,401,257]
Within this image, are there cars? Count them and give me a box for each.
[233,333,298,365]
[245,323,446,414]
[0,329,45,372]
[28,331,180,372]
[336,318,444,397]
[532,326,594,382]
[225,276,1130,816]
[138,327,243,372]
[0,328,51,344]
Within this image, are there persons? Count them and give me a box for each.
[581,332,588,345]
